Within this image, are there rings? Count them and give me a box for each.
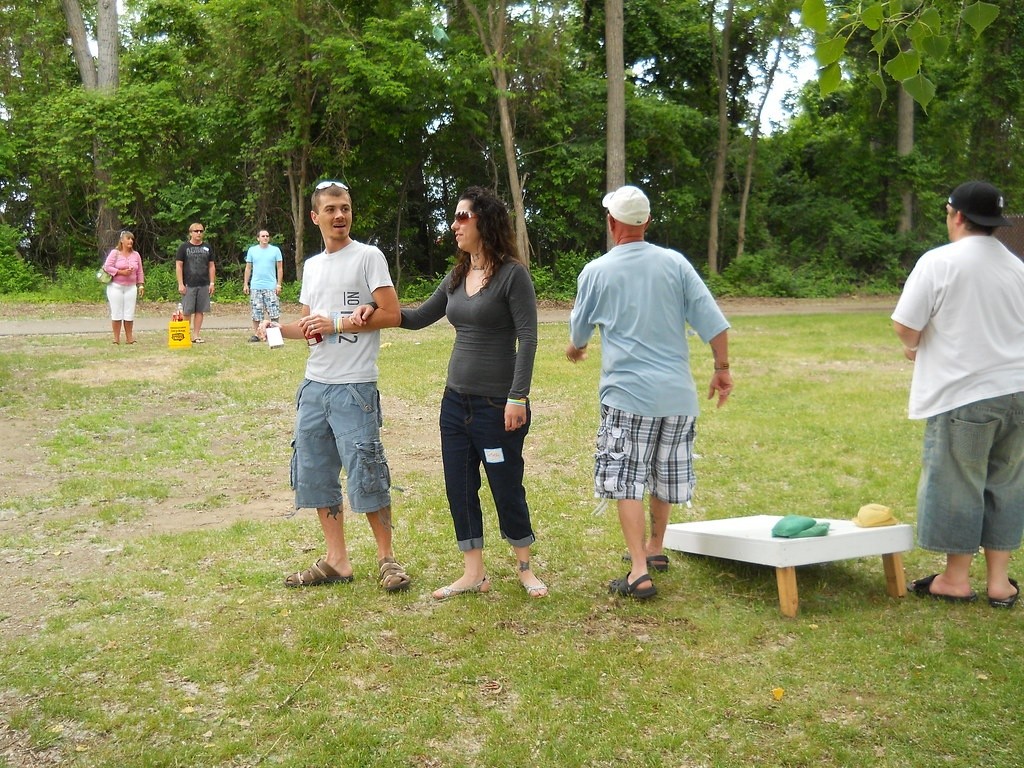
[311,324,314,329]
[518,416,522,424]
[351,313,353,316]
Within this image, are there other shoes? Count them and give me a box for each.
[247,336,261,343]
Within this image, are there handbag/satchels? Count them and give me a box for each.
[97,265,112,284]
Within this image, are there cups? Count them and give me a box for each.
[300,316,322,346]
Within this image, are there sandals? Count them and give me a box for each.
[514,566,549,599]
[621,553,670,572]
[432,574,490,602]
[608,570,658,603]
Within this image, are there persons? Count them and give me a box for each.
[176,223,215,343]
[104,232,144,345]
[258,184,411,593]
[891,182,1024,608]
[566,186,734,598]
[244,230,283,342]
[349,188,548,601]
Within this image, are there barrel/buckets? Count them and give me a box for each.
[168,320,193,349]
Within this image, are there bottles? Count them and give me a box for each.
[172,312,176,321]
[176,302,183,321]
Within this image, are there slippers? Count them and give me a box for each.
[284,557,354,588]
[986,577,1020,610]
[191,337,205,343]
[125,340,138,345]
[906,573,978,603]
[376,556,411,590]
[112,341,120,345]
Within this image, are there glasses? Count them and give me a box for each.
[261,235,269,238]
[454,211,478,223]
[314,181,348,197]
[190,229,204,233]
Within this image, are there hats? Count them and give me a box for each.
[851,503,898,528]
[602,185,651,226]
[947,180,1013,228]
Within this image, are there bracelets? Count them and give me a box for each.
[277,285,282,287]
[507,399,526,406]
[210,283,214,285]
[907,347,918,351]
[714,363,729,370]
[139,286,144,288]
[333,317,344,334]
